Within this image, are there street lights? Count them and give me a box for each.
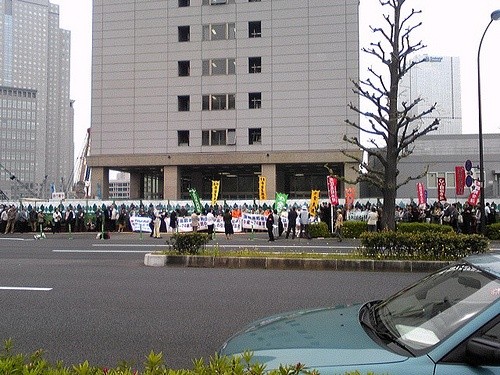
[474,9,499,240]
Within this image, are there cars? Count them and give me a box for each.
[218,253,500,374]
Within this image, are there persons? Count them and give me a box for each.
[285,206,297,239]
[394,200,496,234]
[169,208,180,234]
[335,209,343,242]
[206,209,216,241]
[0,204,383,233]
[367,207,378,232]
[223,209,234,240]
[149,208,169,239]
[299,205,310,238]
[52,208,62,234]
[266,209,275,242]
[273,209,280,240]
[3,205,16,235]
[190,210,199,232]
